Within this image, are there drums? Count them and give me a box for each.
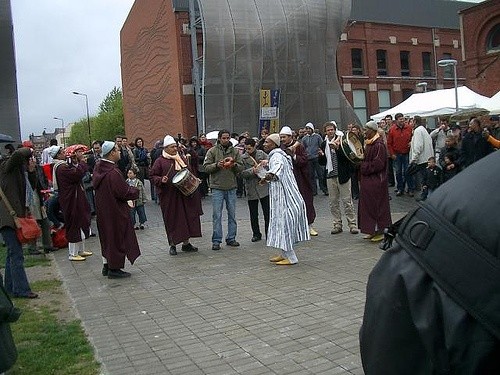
[339,130,366,165]
[172,168,201,196]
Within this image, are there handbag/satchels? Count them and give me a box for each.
[13,215,40,243]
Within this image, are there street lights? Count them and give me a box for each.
[53,117,66,150]
[417,82,428,92]
[73,92,91,148]
[436,60,459,113]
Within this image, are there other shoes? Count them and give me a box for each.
[269,255,284,262]
[102,268,108,276]
[331,227,342,234]
[182,243,198,252]
[324,191,329,195]
[212,244,220,249]
[108,269,131,279]
[350,226,358,234]
[310,228,318,236]
[26,292,37,298]
[275,259,294,265]
[363,233,384,241]
[170,246,177,255]
[314,192,317,196]
[388,184,424,204]
[69,255,86,261]
[79,252,92,256]
[251,235,260,241]
[228,241,239,246]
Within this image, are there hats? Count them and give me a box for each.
[365,121,378,131]
[280,126,292,135]
[163,135,176,147]
[268,134,280,147]
[47,146,60,157]
[102,140,115,155]
[305,123,314,132]
[450,124,461,130]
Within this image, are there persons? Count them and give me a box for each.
[93,141,141,279]
[202,130,243,250]
[49,145,93,261]
[0,271,22,375]
[258,134,311,264]
[318,123,359,234]
[0,136,149,253]
[198,121,390,198]
[357,121,392,241]
[359,148,500,375]
[150,134,204,255]
[150,134,206,204]
[380,113,500,206]
[239,138,270,242]
[280,126,318,236]
[0,147,38,298]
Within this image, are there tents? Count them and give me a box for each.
[371,85,500,120]
